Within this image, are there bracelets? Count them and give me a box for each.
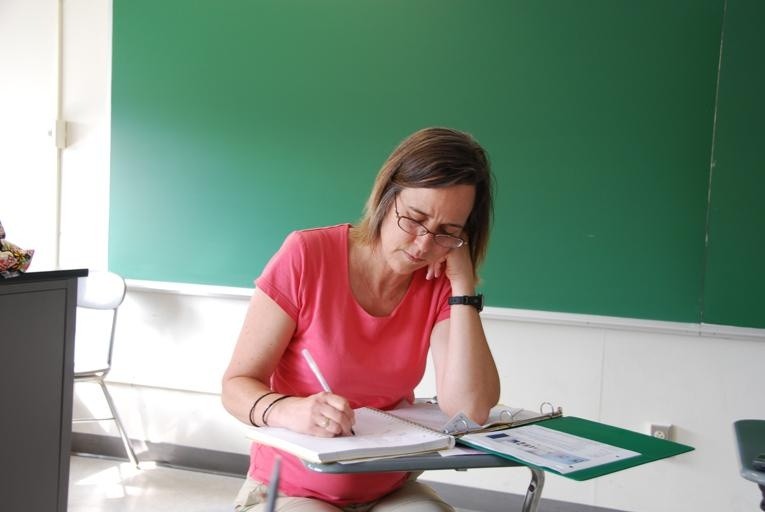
[249,393,276,428]
[261,393,292,428]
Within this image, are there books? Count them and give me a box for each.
[244,407,457,463]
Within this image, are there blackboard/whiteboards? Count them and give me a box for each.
[107,0,765,343]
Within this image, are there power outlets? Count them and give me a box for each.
[650,423,672,441]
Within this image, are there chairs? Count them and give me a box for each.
[73,268,140,469]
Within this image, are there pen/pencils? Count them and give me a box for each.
[302,349,356,436]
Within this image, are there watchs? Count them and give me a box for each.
[448,294,484,313]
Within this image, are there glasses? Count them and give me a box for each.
[390,190,469,250]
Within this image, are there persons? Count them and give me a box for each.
[221,126,500,512]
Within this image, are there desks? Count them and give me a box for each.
[733,419,765,512]
[0,268,88,512]
[263,440,546,512]
